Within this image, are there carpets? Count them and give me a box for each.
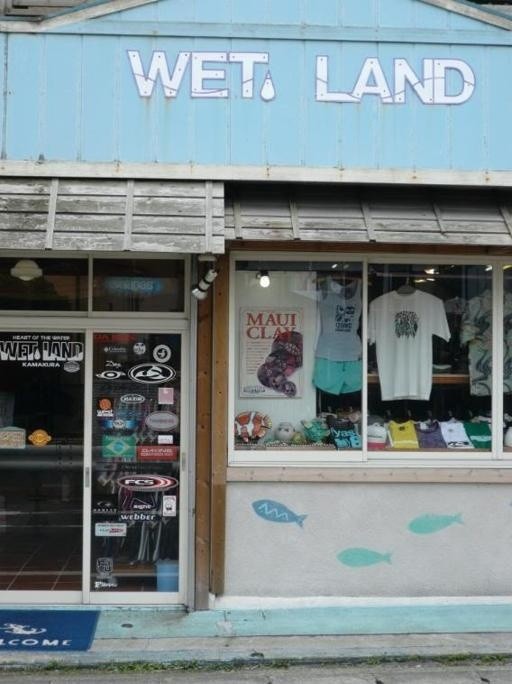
[0,608,100,651]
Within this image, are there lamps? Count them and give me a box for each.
[191,270,220,300]
[259,271,269,288]
[11,258,42,281]
